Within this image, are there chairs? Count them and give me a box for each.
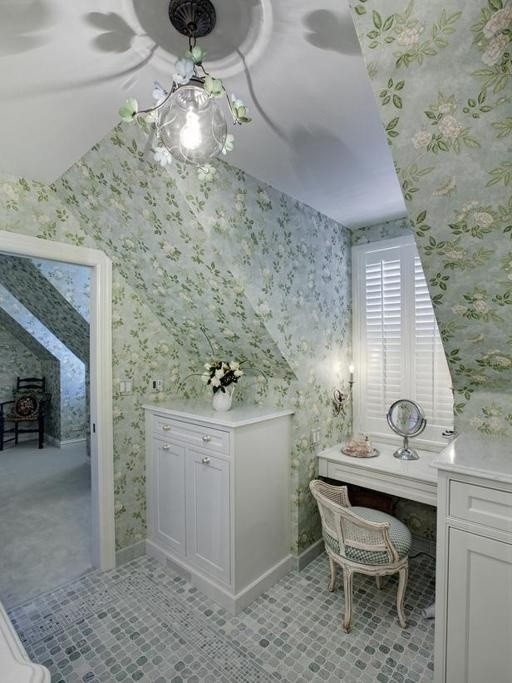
[0,373,47,451]
[308,477,414,634]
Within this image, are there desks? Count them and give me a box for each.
[318,437,436,507]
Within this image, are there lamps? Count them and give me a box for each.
[120,0,254,184]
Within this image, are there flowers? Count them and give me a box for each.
[203,357,245,393]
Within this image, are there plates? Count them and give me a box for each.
[341,445,380,459]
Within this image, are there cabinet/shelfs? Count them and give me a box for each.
[430,428,512,683]
[140,397,294,616]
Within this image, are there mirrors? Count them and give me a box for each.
[385,397,426,460]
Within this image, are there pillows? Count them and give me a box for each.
[7,388,52,423]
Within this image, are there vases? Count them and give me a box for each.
[211,386,233,411]
